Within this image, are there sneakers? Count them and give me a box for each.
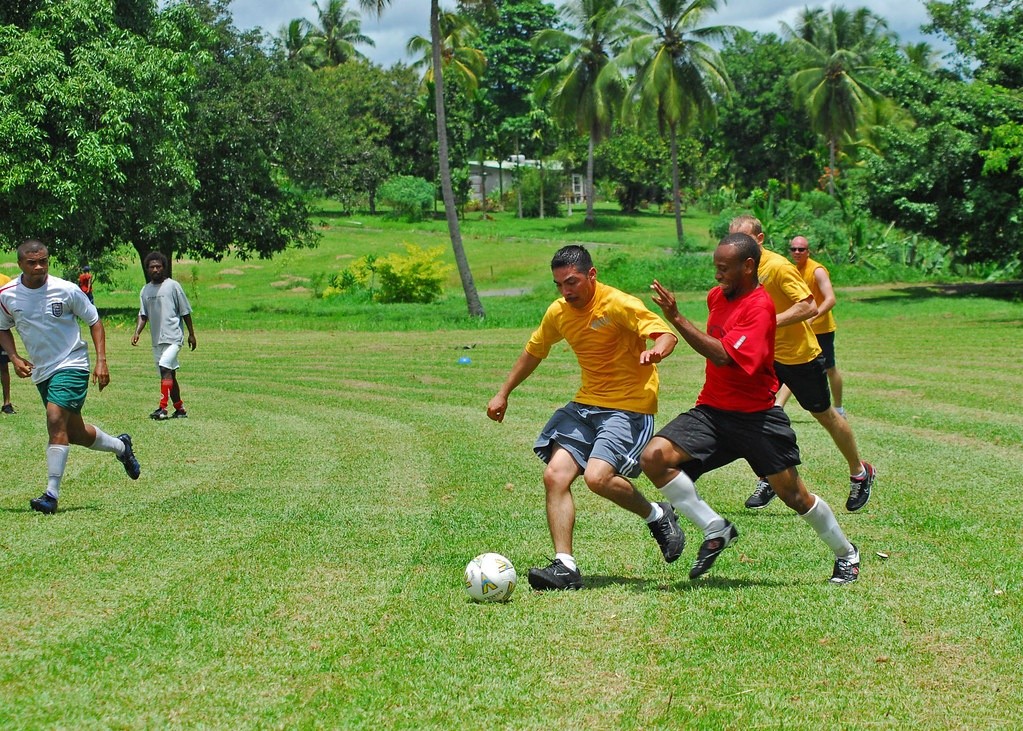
[529,555,583,591]
[650,500,685,564]
[2,403,16,414]
[150,408,168,420]
[116,433,140,480]
[170,411,187,419]
[745,475,777,508]
[846,462,876,511]
[830,543,860,584]
[31,492,58,515]
[690,518,740,581]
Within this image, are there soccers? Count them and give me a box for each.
[464,552,517,602]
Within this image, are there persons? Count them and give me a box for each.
[486,245,685,591]
[771,236,846,424]
[0,239,140,514]
[727,214,875,512]
[640,233,860,587]
[79,266,94,305]
[0,272,21,415]
[131,252,196,420]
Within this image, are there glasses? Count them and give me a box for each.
[790,247,807,252]
